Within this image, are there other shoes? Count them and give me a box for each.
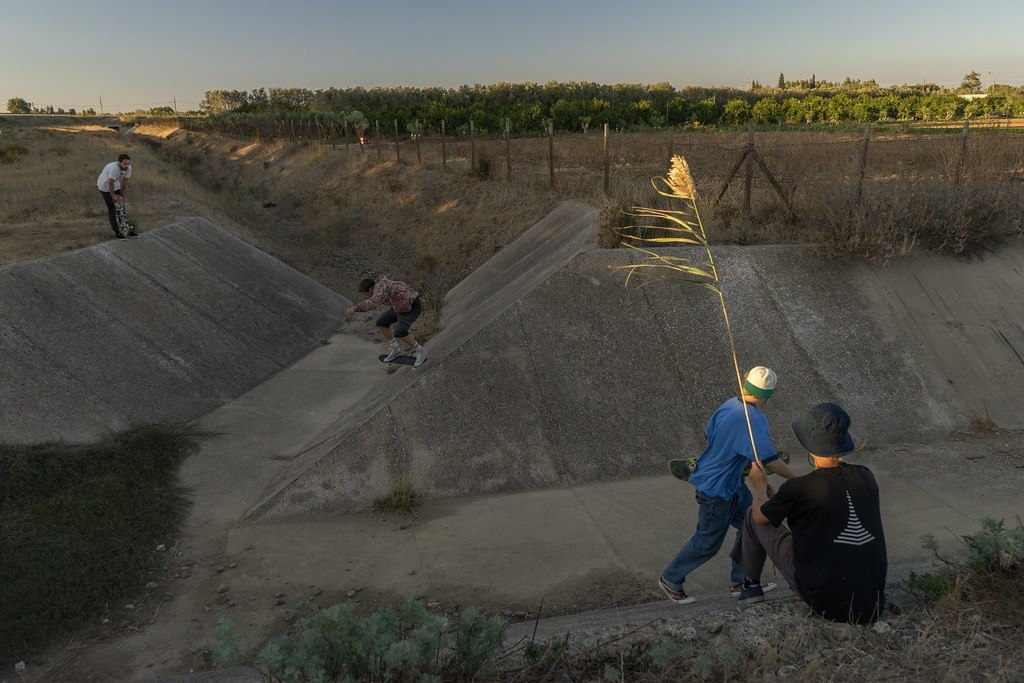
[128,232,140,237]
[658,576,685,602]
[737,585,765,605]
[116,233,127,240]
[730,582,777,597]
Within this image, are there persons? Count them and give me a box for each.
[657,365,796,606]
[96,154,141,240]
[344,278,428,368]
[736,403,887,624]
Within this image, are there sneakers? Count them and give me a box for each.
[384,345,405,363]
[414,348,427,367]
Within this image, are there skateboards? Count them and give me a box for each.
[112,197,133,237]
[668,451,790,481]
[379,355,429,371]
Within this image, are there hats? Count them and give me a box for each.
[789,403,855,457]
[744,366,777,398]
